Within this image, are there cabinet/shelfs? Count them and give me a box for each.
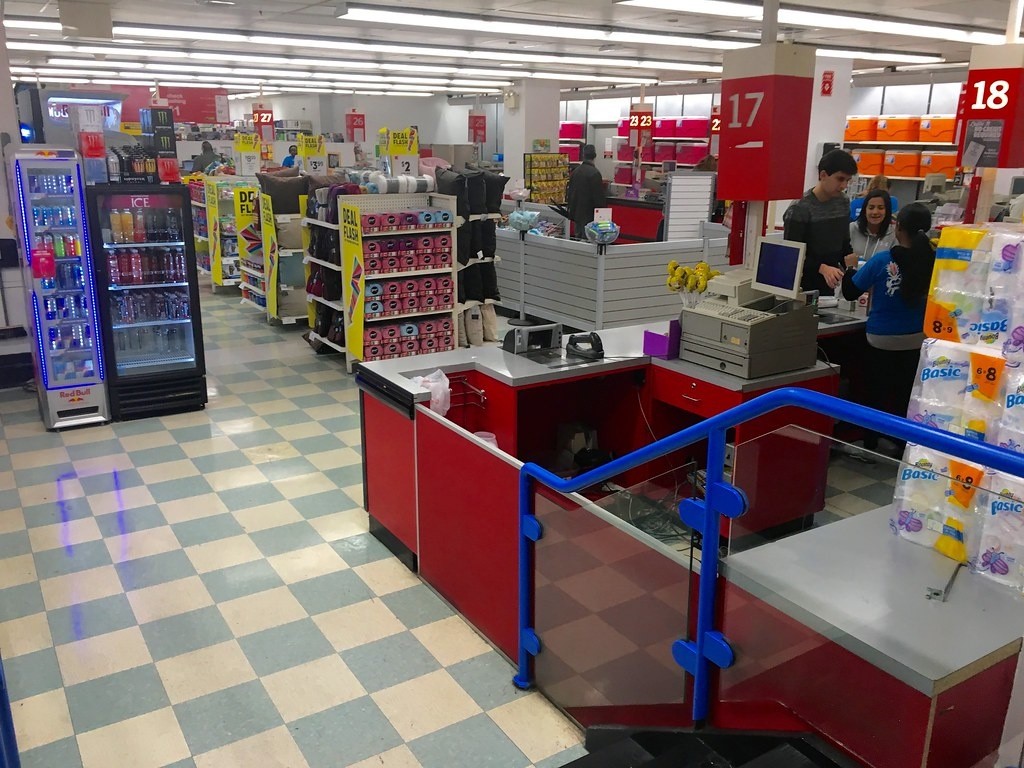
[524,149,570,203]
[305,198,346,363]
[431,191,502,349]
[841,137,962,184]
[182,172,301,378]
[611,135,709,197]
[340,193,461,369]
[560,139,586,165]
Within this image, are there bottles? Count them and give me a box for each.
[112,289,191,353]
[473,431,498,449]
[109,207,182,243]
[106,247,187,286]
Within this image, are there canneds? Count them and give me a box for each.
[109,288,190,321]
[27,174,94,351]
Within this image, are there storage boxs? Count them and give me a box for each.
[875,115,918,142]
[654,141,675,162]
[845,113,876,142]
[919,114,957,141]
[616,141,654,162]
[559,143,581,162]
[852,148,885,176]
[558,120,584,139]
[360,211,455,363]
[83,156,109,184]
[884,150,920,176]
[77,133,105,156]
[139,108,175,132]
[142,132,178,156]
[157,158,182,182]
[674,117,710,138]
[617,118,630,136]
[653,116,675,136]
[919,151,958,177]
[67,104,103,131]
[676,142,708,164]
[614,165,632,184]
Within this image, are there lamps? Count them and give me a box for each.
[3,0,1024,96]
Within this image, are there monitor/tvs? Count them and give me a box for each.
[750,235,807,300]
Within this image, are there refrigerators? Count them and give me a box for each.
[8,143,113,432]
[85,178,210,423]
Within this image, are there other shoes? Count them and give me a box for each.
[848,448,876,464]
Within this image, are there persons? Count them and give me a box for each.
[784,149,857,297]
[568,144,607,241]
[850,176,898,222]
[191,141,221,174]
[694,155,725,221]
[850,190,899,266]
[832,202,937,462]
[282,145,298,169]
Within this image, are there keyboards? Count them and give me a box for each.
[682,298,776,326]
